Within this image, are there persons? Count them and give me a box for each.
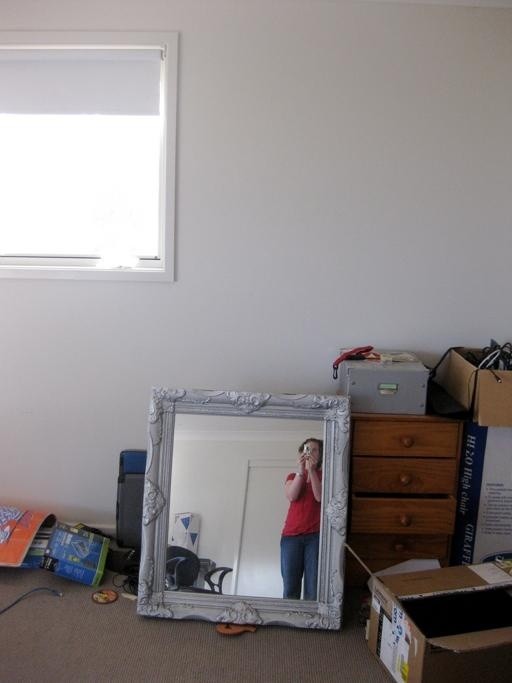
[279,435,323,602]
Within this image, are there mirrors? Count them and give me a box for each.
[133,383,355,635]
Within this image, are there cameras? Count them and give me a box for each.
[303,444,312,454]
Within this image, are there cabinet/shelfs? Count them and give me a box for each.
[344,411,466,602]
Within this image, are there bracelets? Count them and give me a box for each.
[295,471,304,478]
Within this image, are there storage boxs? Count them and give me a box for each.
[362,555,512,682]
[445,343,509,432]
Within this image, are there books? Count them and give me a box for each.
[0,503,58,570]
